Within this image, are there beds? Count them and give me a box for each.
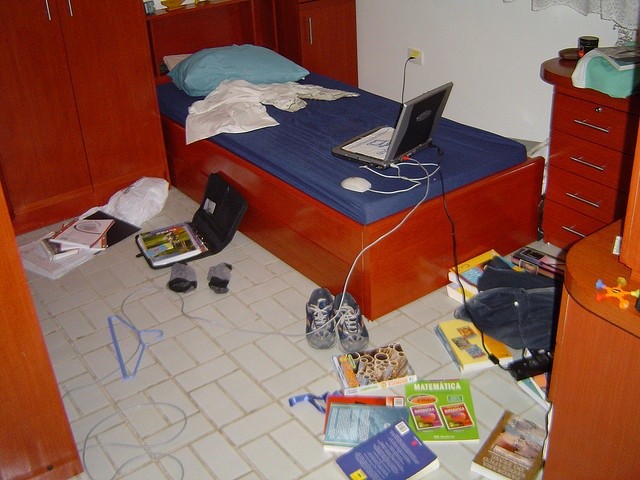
[157,73,546,322]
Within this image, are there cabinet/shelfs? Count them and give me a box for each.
[279,0,358,89]
[0,0,172,239]
[541,45,639,252]
[143,0,278,73]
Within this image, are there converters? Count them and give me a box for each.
[507,352,553,382]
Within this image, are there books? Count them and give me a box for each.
[518,377,547,410]
[324,406,410,451]
[323,395,404,439]
[448,284,476,306]
[595,46,637,85]
[470,408,547,480]
[134,222,208,266]
[335,421,440,480]
[332,342,416,394]
[449,249,524,293]
[48,216,115,243]
[59,234,105,252]
[436,319,513,372]
[35,239,84,259]
[522,348,540,362]
[530,372,547,396]
[405,378,480,450]
[476,249,559,280]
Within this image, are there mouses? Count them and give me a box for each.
[341,177,370,194]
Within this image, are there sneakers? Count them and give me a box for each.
[305,286,337,351]
[334,291,370,354]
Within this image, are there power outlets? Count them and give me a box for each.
[407,48,422,65]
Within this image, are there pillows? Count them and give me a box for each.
[167,43,310,98]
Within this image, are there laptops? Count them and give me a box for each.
[332,83,453,166]
